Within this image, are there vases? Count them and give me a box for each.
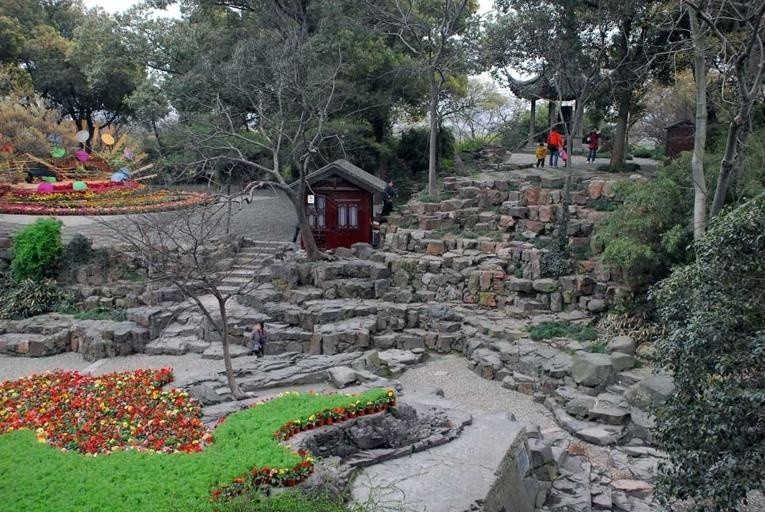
[213,398,400,486]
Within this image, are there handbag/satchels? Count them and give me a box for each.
[586,135,592,144]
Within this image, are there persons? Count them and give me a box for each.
[291,222,302,242]
[381,177,397,218]
[585,126,602,164]
[547,125,564,168]
[562,142,571,167]
[533,142,546,168]
[250,318,268,359]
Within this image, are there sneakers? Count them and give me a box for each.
[536,160,597,169]
